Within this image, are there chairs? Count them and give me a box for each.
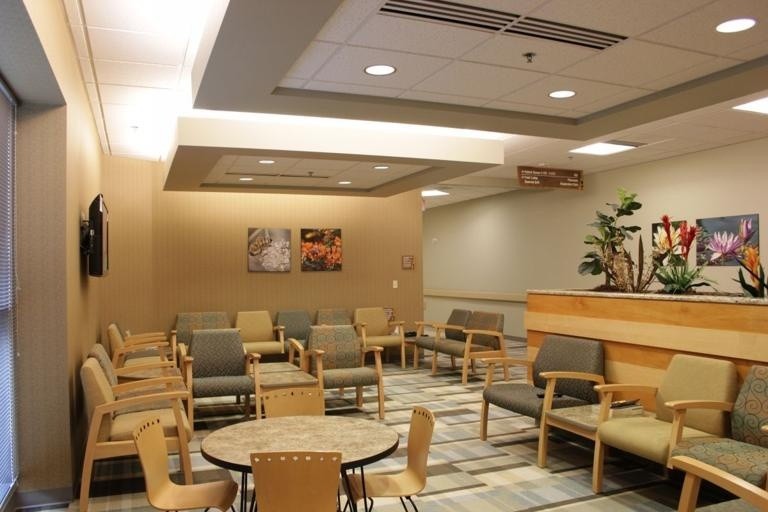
[77,322,197,512]
[479,333,608,470]
[354,307,406,371]
[412,308,511,386]
[131,415,239,512]
[250,452,344,512]
[235,310,287,357]
[662,364,768,512]
[307,320,387,421]
[181,329,261,420]
[174,310,233,369]
[262,385,326,420]
[545,399,658,441]
[345,404,434,512]
[314,306,350,327]
[277,307,307,357]
[590,353,738,497]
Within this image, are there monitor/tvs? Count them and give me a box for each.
[89,194,109,277]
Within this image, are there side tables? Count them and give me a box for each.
[248,353,319,419]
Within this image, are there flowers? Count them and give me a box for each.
[733,245,766,299]
[705,216,755,262]
[648,212,718,287]
[651,223,687,267]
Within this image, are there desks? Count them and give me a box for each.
[200,414,400,512]
